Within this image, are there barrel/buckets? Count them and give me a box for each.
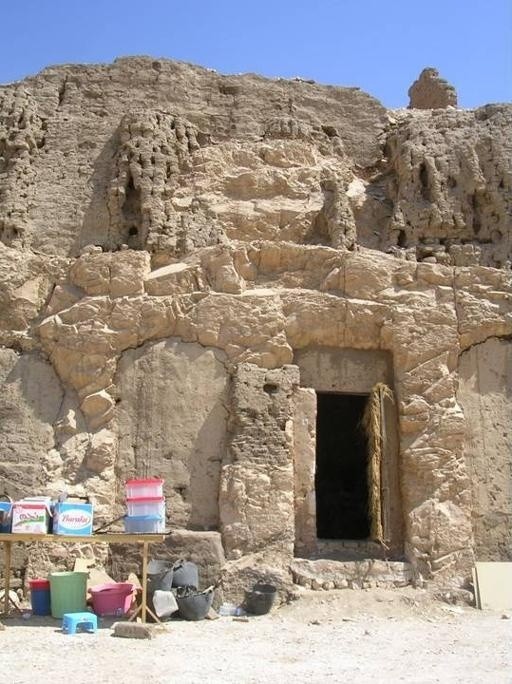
[49,571,91,618]
[244,580,277,615]
[147,559,174,589]
[173,562,199,588]
[29,579,50,589]
[31,587,51,616]
[176,586,215,622]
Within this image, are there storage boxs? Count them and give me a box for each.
[53,500,93,536]
[125,516,165,531]
[11,500,52,534]
[126,478,163,498]
[127,498,165,516]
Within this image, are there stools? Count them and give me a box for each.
[62,612,97,634]
